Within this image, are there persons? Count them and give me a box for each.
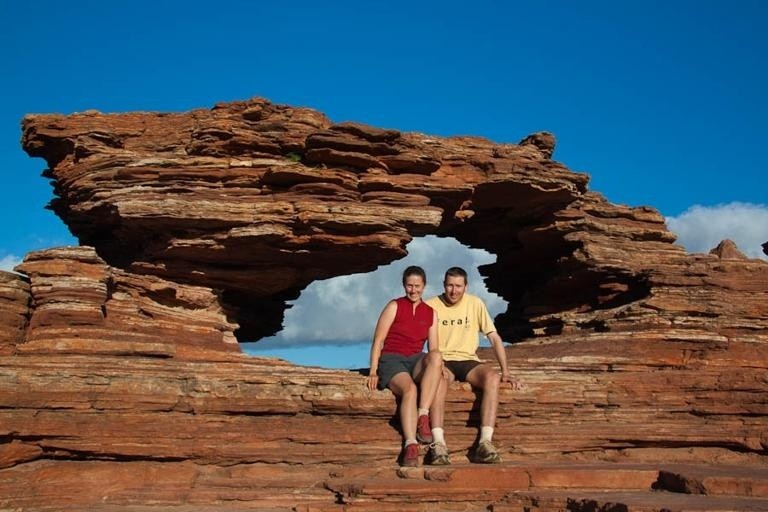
[366,262,443,467]
[423,264,525,466]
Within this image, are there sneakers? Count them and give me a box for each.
[475,441,502,462]
[416,415,432,443]
[399,444,419,468]
[429,443,450,464]
